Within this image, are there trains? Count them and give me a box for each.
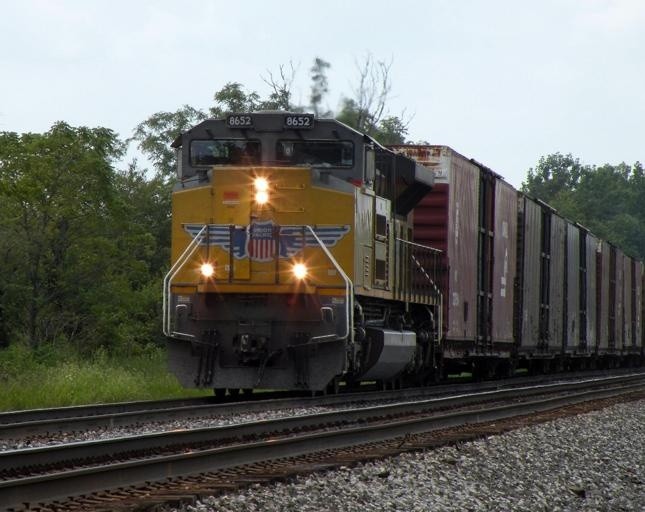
[159,111,645,397]
